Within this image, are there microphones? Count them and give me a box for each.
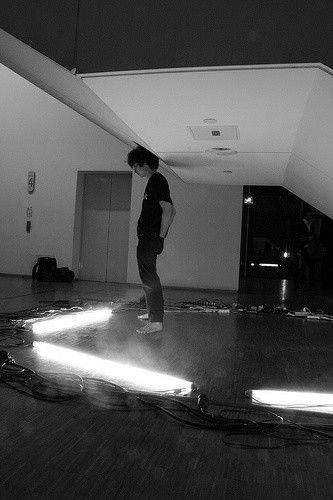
[197,393,208,411]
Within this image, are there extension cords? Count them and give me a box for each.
[284,310,311,317]
[189,304,229,313]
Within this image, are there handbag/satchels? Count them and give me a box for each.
[54,267,74,281]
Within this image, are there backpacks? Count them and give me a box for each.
[32,257,56,282]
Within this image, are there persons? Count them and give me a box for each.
[127,141,176,334]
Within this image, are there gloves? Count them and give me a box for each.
[153,237,164,254]
